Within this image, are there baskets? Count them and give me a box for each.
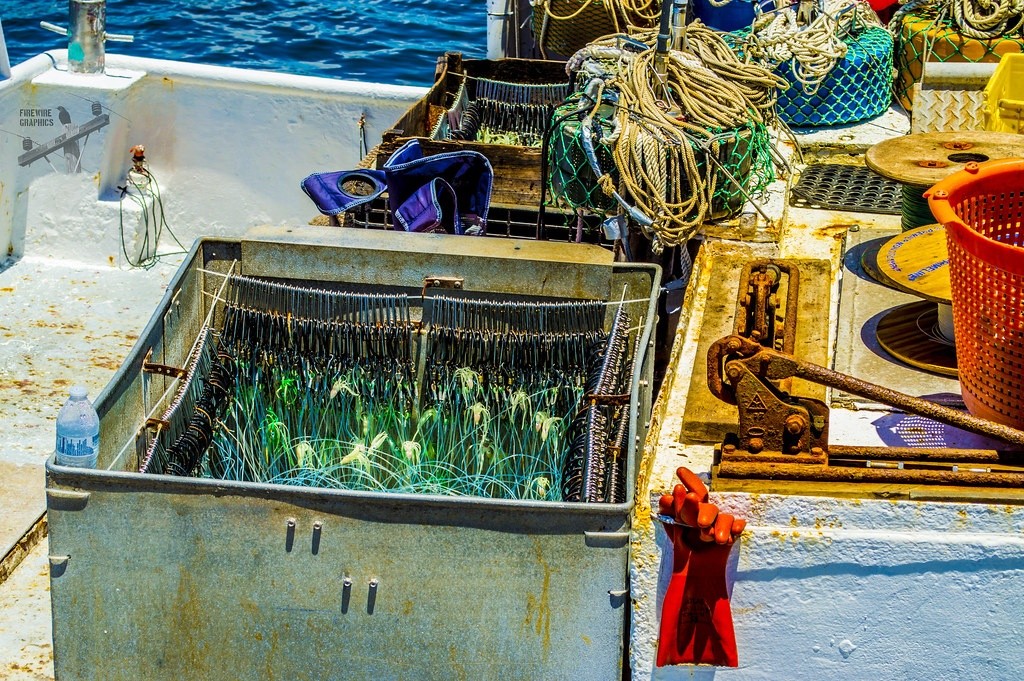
[922,159,1024,436]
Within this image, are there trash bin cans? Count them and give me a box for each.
[922,158,1024,445]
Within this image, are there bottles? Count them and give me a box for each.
[54,385,101,469]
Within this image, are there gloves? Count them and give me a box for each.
[660,467,733,545]
[657,485,746,668]
